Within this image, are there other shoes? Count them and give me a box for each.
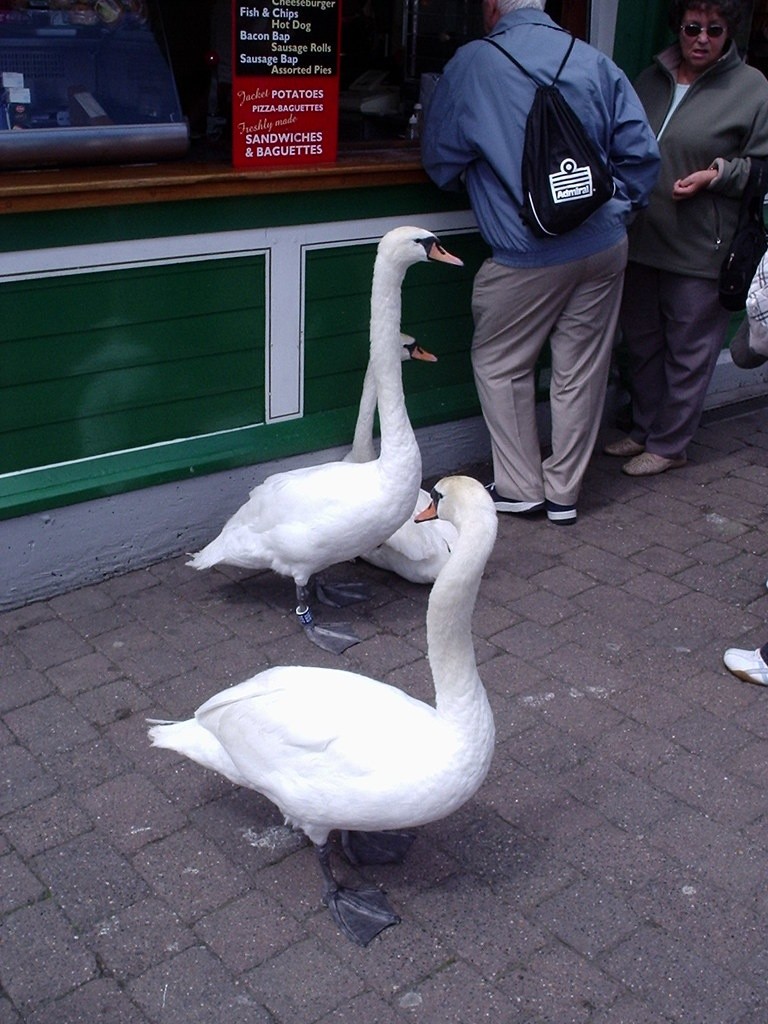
[485,482,545,516]
[544,495,577,526]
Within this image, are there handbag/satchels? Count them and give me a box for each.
[716,158,768,311]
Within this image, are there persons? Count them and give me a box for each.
[427,0,659,525]
[724,191,768,687]
[603,0,768,475]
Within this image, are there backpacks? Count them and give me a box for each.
[481,33,621,239]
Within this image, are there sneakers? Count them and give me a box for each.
[622,451,687,476]
[605,438,648,456]
[723,647,768,687]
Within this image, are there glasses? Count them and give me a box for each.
[678,24,729,37]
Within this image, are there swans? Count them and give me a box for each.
[146,475,499,948]
[182,224,465,660]
